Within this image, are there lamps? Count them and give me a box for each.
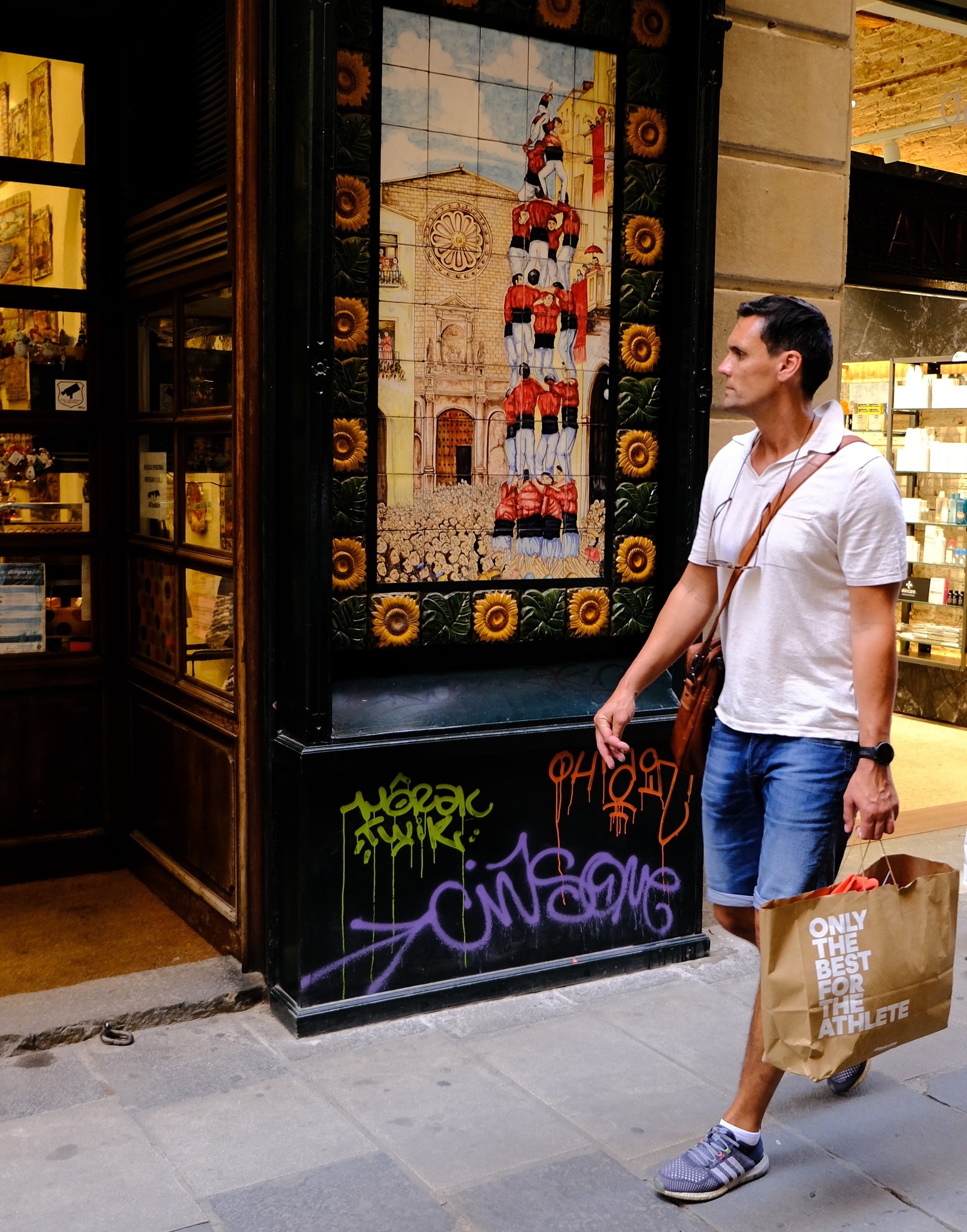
[882,139,901,164]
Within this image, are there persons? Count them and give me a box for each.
[593,295,907,1202]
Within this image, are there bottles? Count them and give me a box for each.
[958,591,964,606]
[945,539,955,564]
[935,491,965,525]
[947,590,953,605]
[952,548,961,565]
[921,500,929,522]
[953,590,959,605]
[959,548,966,565]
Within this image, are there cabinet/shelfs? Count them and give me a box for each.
[885,355,967,672]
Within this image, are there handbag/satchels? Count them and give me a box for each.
[673,636,725,777]
[760,829,962,1079]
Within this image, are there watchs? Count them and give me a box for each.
[854,743,896,769]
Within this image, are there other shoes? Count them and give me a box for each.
[653,1124,770,1200]
[826,1058,870,1092]
